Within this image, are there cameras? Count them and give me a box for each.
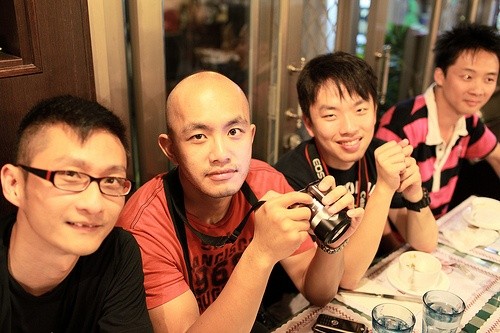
[287,179,352,244]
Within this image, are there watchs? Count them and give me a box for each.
[403,188,431,212]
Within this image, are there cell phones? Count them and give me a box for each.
[311,314,369,333]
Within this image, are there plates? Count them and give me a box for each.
[461,207,500,231]
[386,261,450,296]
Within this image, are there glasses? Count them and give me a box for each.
[15,162,135,197]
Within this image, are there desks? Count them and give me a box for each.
[273,193,500,333]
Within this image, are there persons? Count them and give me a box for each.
[0,94,153,333]
[272,51,439,290]
[115,71,365,333]
[374,21,500,250]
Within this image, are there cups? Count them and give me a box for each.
[421,290,465,333]
[372,303,416,333]
[470,197,500,227]
[399,250,441,290]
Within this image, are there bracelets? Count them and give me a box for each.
[315,237,349,255]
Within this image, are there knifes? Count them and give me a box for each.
[339,285,421,304]
[475,245,497,255]
